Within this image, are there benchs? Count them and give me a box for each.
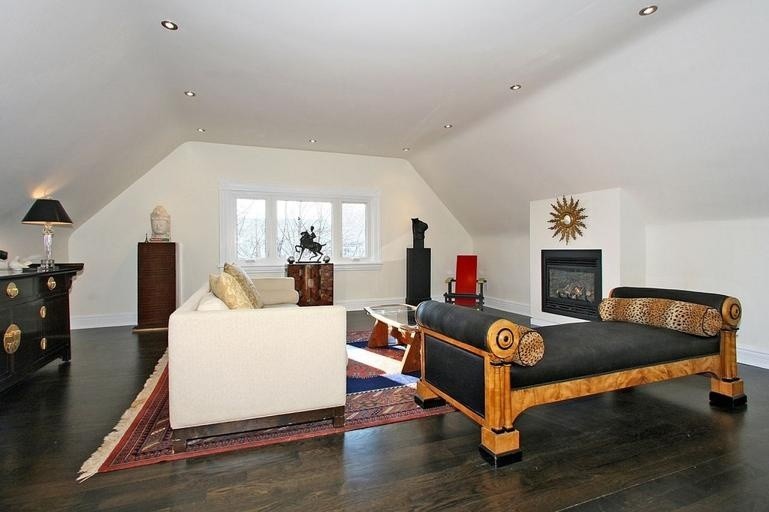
[413,286,748,468]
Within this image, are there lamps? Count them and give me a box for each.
[21,198,73,271]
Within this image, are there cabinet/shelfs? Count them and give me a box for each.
[288,262,333,306]
[0,261,84,394]
[137,242,176,333]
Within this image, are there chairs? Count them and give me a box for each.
[444,255,486,312]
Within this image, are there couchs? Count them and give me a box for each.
[166,275,350,455]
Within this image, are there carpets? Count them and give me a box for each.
[75,326,458,484]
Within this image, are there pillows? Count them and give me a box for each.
[598,297,724,339]
[225,264,264,308]
[207,271,251,310]
[195,293,227,310]
[511,325,544,367]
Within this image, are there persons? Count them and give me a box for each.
[309,226,315,241]
[150,205,171,241]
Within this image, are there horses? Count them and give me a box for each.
[295,230,326,262]
